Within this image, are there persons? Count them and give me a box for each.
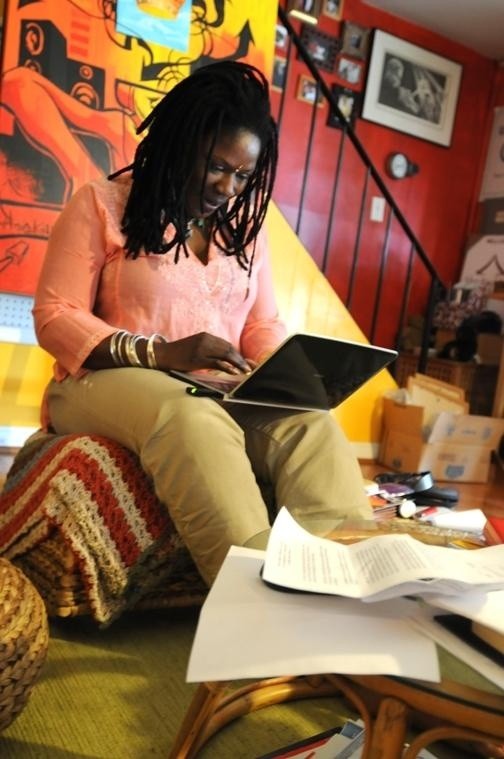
[30,62,375,593]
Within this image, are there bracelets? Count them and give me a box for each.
[109,329,167,369]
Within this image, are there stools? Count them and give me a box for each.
[10,433,212,630]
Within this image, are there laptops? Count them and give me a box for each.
[169,334,399,414]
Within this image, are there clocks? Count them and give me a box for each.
[386,153,421,181]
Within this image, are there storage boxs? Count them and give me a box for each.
[376,396,504,484]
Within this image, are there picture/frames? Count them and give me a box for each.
[361,27,466,148]
[272,0,367,132]
